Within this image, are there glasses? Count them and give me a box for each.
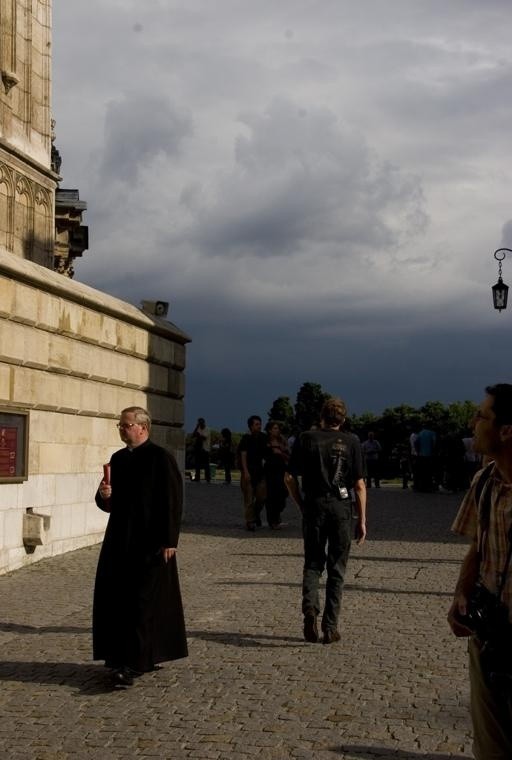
[117,422,140,429]
[474,411,492,423]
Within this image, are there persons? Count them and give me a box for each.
[93,407,189,689]
[283,398,367,644]
[191,415,480,532]
[447,382,512,760]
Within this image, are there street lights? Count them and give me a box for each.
[491,247,512,314]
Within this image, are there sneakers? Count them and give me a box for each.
[275,522,289,531]
[324,629,340,644]
[303,608,319,642]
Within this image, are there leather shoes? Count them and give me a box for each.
[109,671,133,686]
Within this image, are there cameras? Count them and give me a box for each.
[454,580,497,641]
